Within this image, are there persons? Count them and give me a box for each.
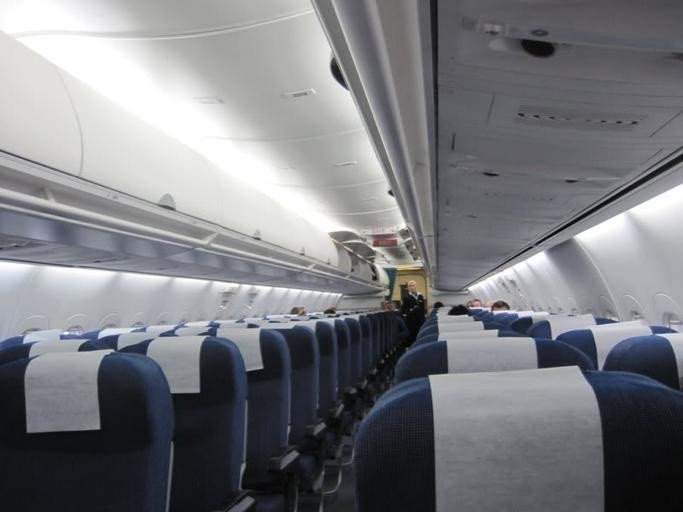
[466,300,472,307]
[448,304,471,316]
[322,308,335,314]
[490,300,509,311]
[432,301,444,309]
[401,279,425,341]
[290,306,307,315]
[471,299,481,308]
